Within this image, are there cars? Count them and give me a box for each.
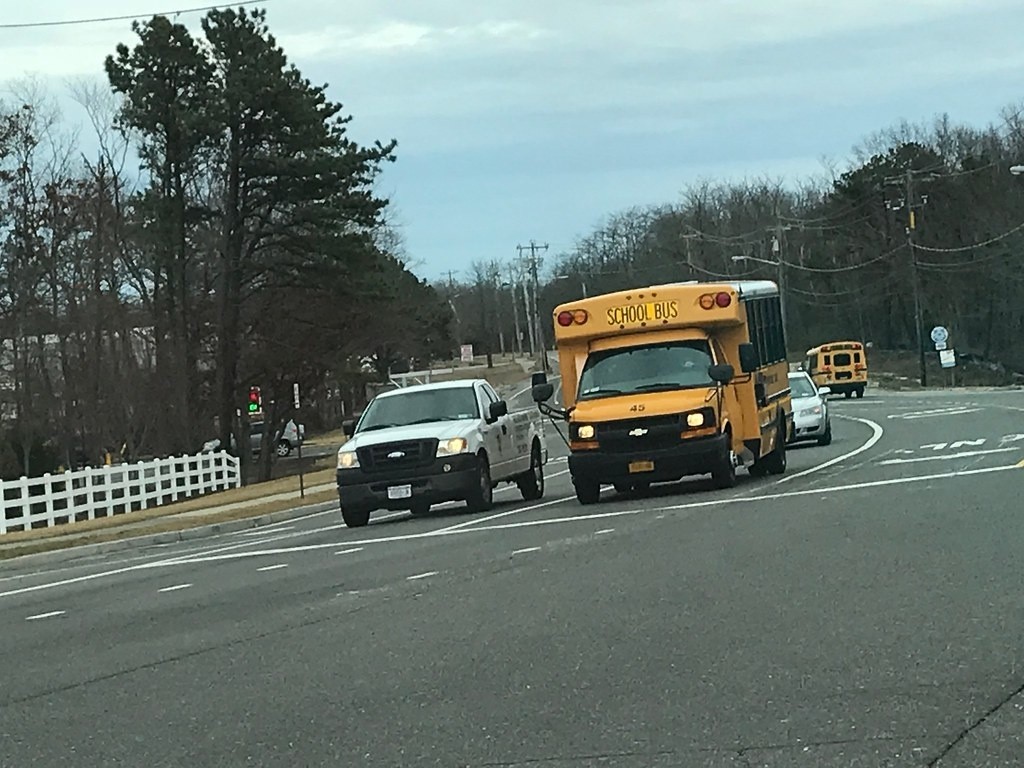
[787,371,833,446]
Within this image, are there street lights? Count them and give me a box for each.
[731,255,790,369]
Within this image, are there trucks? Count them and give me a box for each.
[531,280,797,506]
[806,340,868,399]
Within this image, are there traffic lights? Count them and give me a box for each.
[248,388,258,413]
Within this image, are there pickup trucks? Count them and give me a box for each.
[335,379,550,529]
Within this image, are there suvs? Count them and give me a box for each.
[203,418,307,457]
